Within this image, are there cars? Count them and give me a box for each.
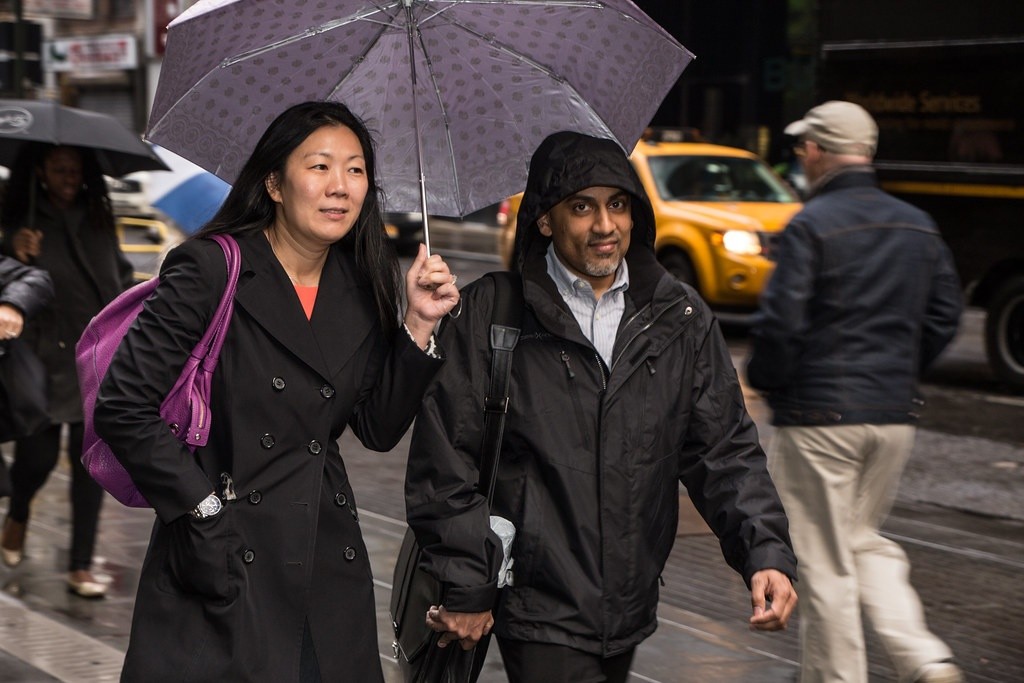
[120,215,185,282]
[498,137,807,307]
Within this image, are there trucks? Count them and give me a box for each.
[816,41,1024,388]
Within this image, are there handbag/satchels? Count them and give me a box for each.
[389,524,507,683]
[74,232,242,509]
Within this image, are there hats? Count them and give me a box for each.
[782,101,879,156]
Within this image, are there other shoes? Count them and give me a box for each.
[0,515,22,566]
[66,575,107,598]
